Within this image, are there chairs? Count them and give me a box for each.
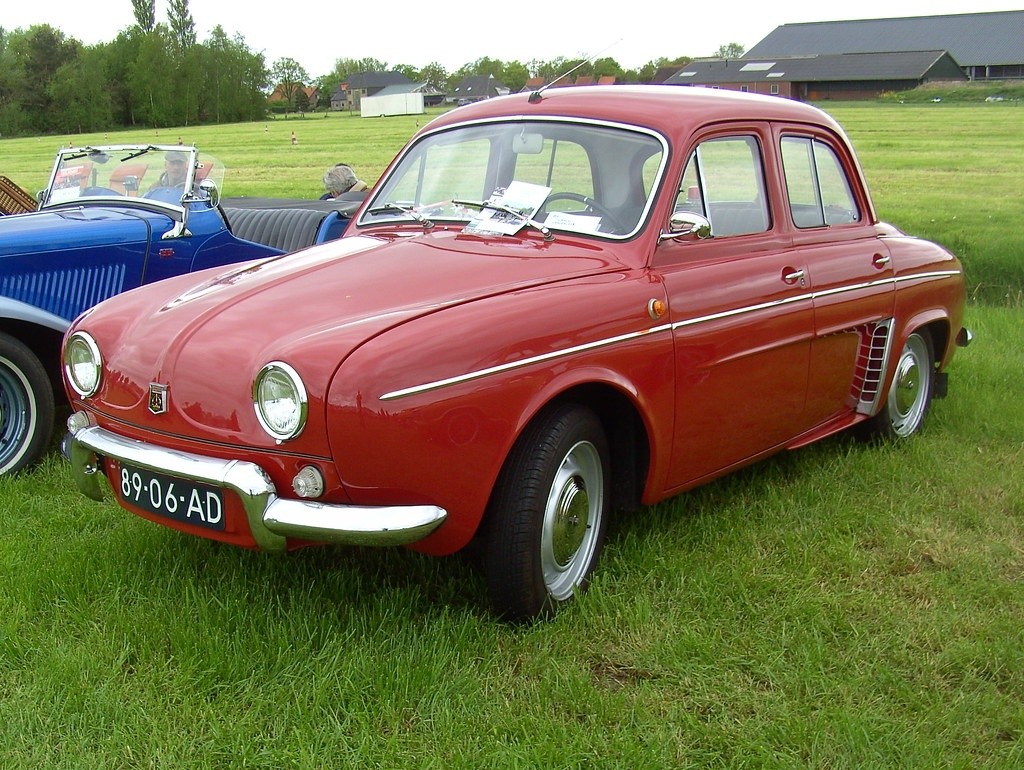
[54,161,93,189]
[108,163,148,198]
[184,162,213,185]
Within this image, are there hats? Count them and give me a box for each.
[164,150,189,163]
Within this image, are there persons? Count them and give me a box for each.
[149,150,198,190]
[320,163,371,200]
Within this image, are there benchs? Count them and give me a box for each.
[670,204,852,236]
[221,207,329,254]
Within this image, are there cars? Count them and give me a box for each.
[0,145,370,477]
[63,38,971,619]
